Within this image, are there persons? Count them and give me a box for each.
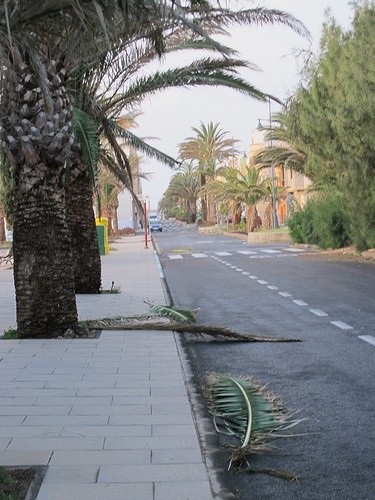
[140,219,144,230]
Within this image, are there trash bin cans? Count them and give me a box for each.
[96,217,108,256]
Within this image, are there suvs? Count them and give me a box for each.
[149,215,160,224]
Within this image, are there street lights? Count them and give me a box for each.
[256,118,278,230]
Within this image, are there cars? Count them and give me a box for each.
[149,220,162,233]
